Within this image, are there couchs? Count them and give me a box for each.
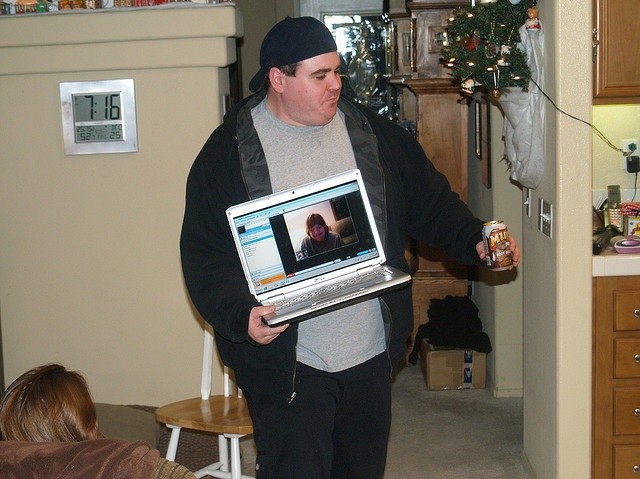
[0,440,198,479]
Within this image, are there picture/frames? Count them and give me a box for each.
[481,95,491,191]
[474,100,482,160]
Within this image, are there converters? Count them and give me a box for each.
[627,155,640,172]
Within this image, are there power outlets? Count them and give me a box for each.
[621,138,640,168]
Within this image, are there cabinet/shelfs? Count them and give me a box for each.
[591,275,640,479]
[593,0,640,104]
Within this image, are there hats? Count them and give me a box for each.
[249,16,337,92]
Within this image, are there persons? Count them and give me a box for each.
[296,213,346,258]
[0,364,103,443]
[179,15,522,477]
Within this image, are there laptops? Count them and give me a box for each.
[225,169,412,327]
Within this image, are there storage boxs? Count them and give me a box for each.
[419,337,486,391]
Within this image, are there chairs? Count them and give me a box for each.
[154,317,257,478]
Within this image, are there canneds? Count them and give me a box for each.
[480,218,515,274]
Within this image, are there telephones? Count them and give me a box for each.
[603,184,624,233]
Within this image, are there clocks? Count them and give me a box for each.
[56,78,139,155]
